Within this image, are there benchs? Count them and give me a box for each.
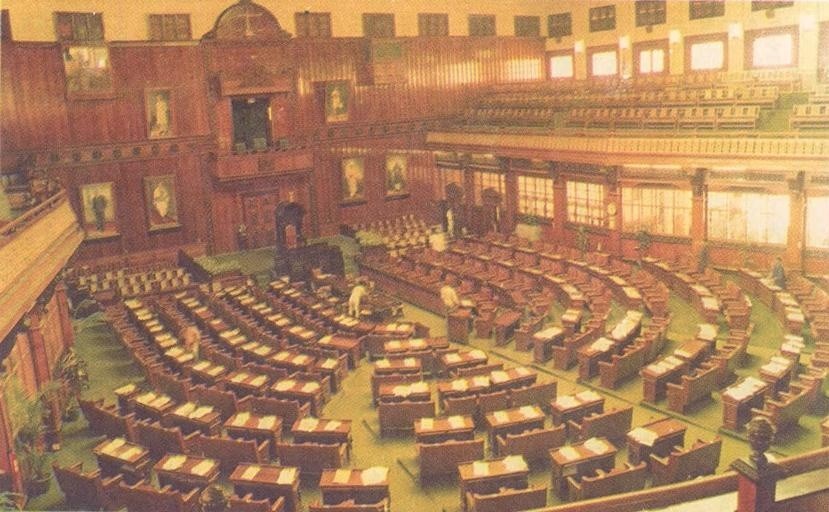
[416,437,484,486]
[463,483,547,512]
[495,422,566,464]
[281,439,346,475]
[119,476,200,511]
[649,435,722,486]
[109,238,829,415]
[309,497,389,512]
[565,458,647,502]
[204,432,272,467]
[568,406,634,438]
[78,397,136,434]
[53,459,121,511]
[132,417,204,454]
[224,491,287,512]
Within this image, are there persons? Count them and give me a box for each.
[768,256,785,289]
[439,282,460,310]
[633,230,651,259]
[156,95,170,135]
[329,86,346,117]
[177,324,201,361]
[92,189,109,232]
[576,227,592,252]
[429,228,447,254]
[348,281,368,318]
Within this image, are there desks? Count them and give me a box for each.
[292,414,353,444]
[154,449,224,491]
[92,436,152,484]
[411,414,474,436]
[547,436,618,487]
[624,417,689,466]
[166,400,221,434]
[456,453,529,511]
[224,411,283,441]
[225,463,300,511]
[484,405,546,448]
[320,466,390,505]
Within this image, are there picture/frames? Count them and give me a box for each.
[77,181,121,242]
[143,87,176,141]
[57,41,119,101]
[143,174,181,233]
[383,151,411,201]
[323,78,347,123]
[338,156,368,206]
